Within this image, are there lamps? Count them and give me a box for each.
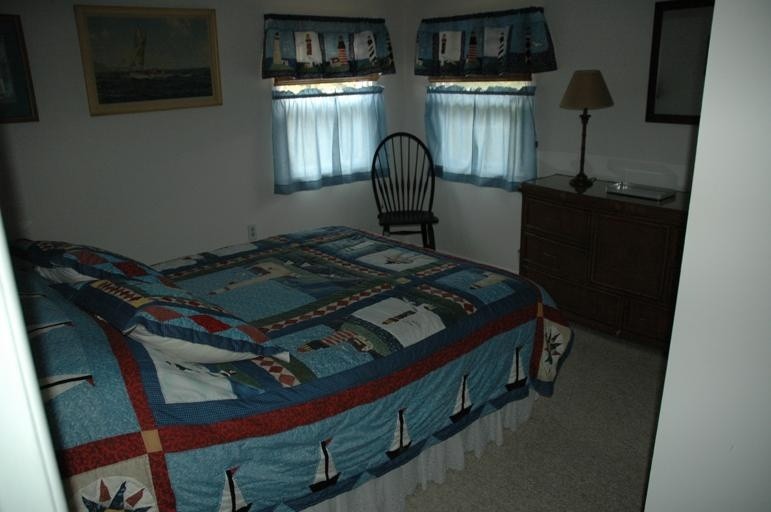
[560,67,617,193]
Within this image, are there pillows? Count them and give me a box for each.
[18,238,291,367]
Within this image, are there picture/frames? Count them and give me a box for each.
[73,5,225,117]
[645,3,715,128]
[1,15,41,122]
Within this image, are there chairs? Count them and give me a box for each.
[371,131,440,256]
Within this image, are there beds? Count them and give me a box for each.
[7,224,550,512]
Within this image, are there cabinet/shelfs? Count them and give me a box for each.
[520,174,693,354]
